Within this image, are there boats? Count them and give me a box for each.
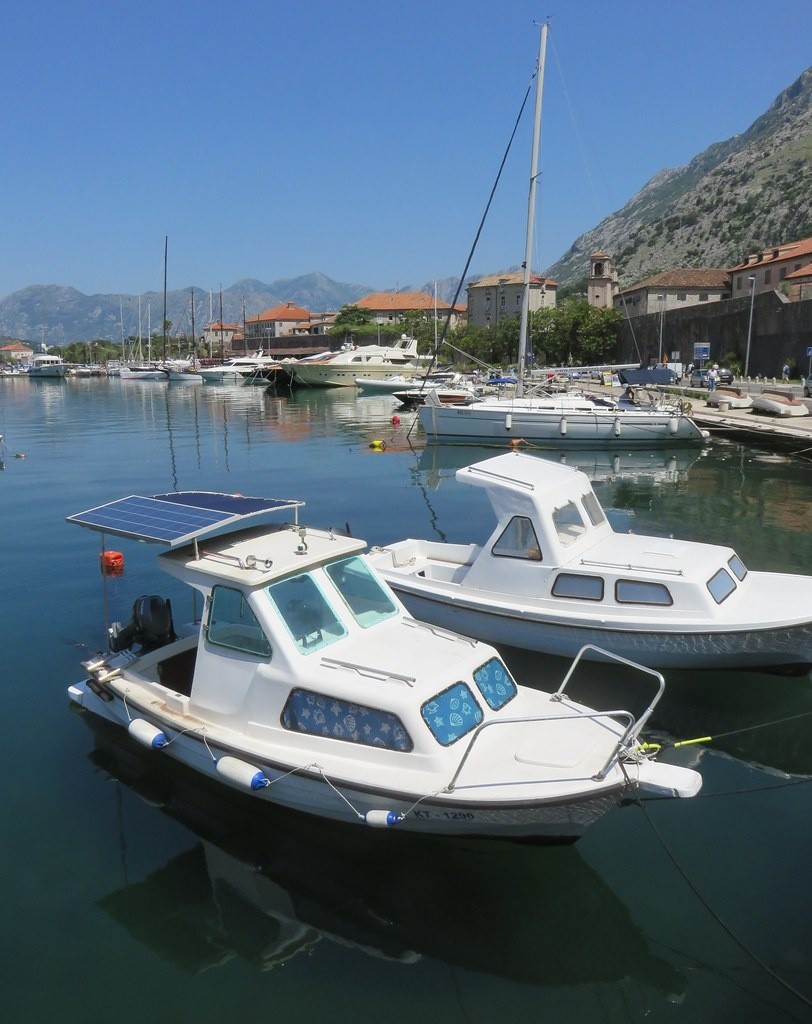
[748,389,810,418]
[66,700,693,1007]
[64,489,705,848]
[0,234,499,412]
[340,449,811,671]
[705,389,754,409]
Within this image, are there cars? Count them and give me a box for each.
[690,367,734,388]
[803,373,812,398]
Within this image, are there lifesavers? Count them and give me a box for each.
[681,401,692,414]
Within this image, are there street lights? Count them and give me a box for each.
[743,275,758,379]
[657,295,665,364]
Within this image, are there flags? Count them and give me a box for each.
[662,351,669,365]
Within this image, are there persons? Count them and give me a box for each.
[781,363,789,384]
[707,366,717,393]
[800,373,806,387]
[687,360,697,376]
[711,362,720,369]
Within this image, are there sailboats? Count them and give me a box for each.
[409,15,711,446]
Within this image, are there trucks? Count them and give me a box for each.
[654,362,683,385]
